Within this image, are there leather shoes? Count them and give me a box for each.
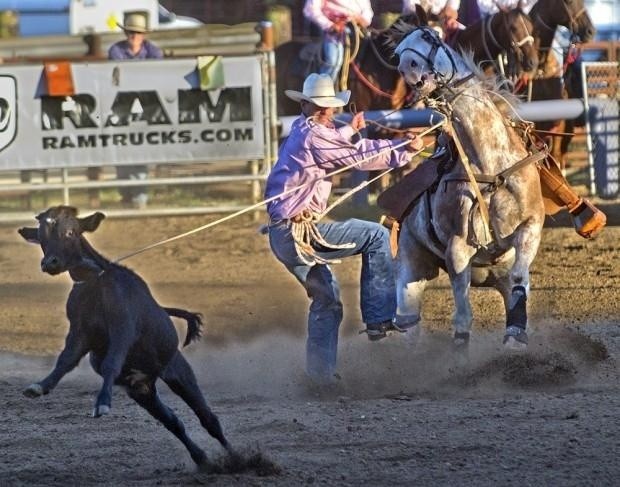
[365,314,421,342]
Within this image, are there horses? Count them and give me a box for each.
[392,0,545,397]
[445,0,540,77]
[274,3,452,193]
[528,0,595,170]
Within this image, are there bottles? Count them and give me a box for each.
[256,19,274,49]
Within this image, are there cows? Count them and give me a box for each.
[17,204,245,470]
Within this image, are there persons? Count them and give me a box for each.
[400,0,462,38]
[301,0,374,82]
[264,71,425,396]
[476,0,539,18]
[107,11,164,209]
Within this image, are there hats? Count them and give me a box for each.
[284,72,353,109]
[117,14,150,34]
